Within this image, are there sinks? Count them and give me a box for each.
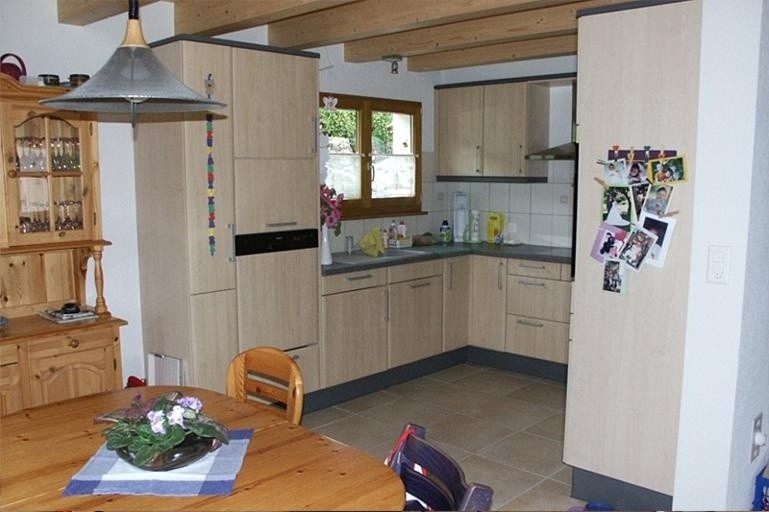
[358,246,427,259]
[336,253,379,264]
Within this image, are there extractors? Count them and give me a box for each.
[524,77,577,161]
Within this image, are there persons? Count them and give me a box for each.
[648,187,670,217]
[615,230,625,242]
[599,232,619,259]
[634,188,644,210]
[608,261,623,295]
[609,191,631,226]
[626,164,642,185]
[653,162,680,183]
[607,164,623,184]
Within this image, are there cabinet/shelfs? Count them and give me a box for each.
[484,83,549,183]
[560,2,705,511]
[0,307,129,415]
[322,247,388,404]
[507,243,572,382]
[233,40,320,421]
[444,238,468,371]
[433,82,481,183]
[468,239,507,370]
[387,246,444,387]
[131,34,238,398]
[1,74,111,253]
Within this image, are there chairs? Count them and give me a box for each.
[226,344,305,426]
[384,422,494,511]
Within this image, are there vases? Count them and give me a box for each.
[116,428,222,471]
[321,217,333,267]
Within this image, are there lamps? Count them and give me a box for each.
[36,1,226,129]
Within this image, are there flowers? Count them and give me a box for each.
[321,182,344,236]
[93,388,231,467]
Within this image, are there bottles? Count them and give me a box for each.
[7,130,83,234]
[381,218,400,249]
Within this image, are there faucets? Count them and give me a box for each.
[343,233,358,255]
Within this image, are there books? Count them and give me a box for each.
[38,312,100,324]
[43,310,99,319]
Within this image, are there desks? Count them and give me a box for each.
[0,383,407,511]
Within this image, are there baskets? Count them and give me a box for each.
[388,238,412,248]
